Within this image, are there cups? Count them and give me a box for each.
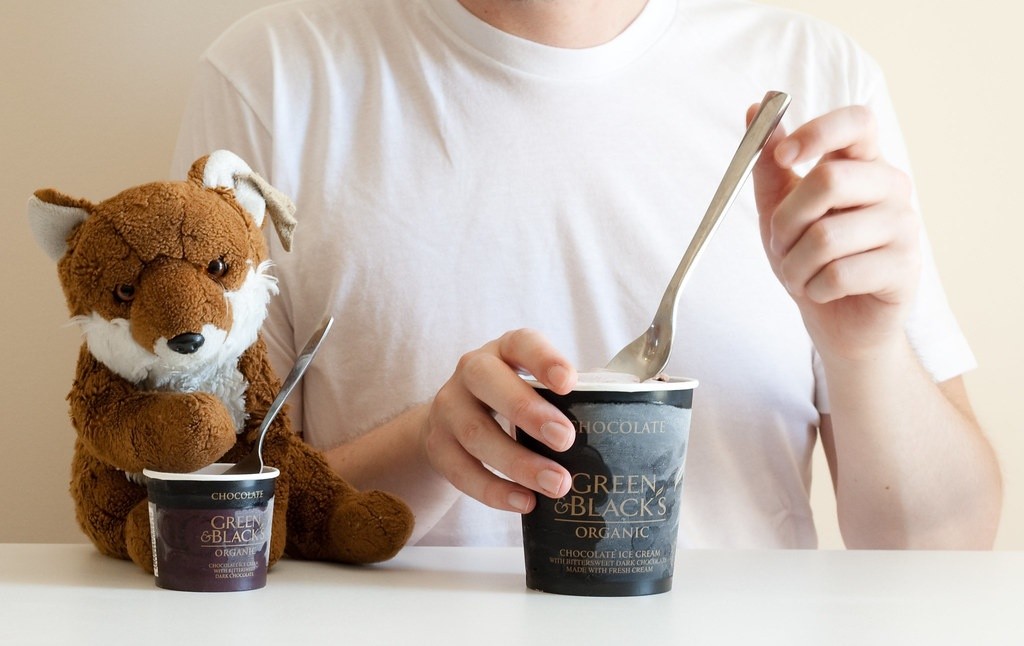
[143,463,279,593]
[510,375,698,599]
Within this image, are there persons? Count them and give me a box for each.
[173,0,1002,552]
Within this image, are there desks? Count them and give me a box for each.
[0,545,1021,644]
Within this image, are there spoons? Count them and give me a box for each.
[218,317,334,476]
[604,89,792,384]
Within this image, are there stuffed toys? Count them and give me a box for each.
[28,149,416,575]
